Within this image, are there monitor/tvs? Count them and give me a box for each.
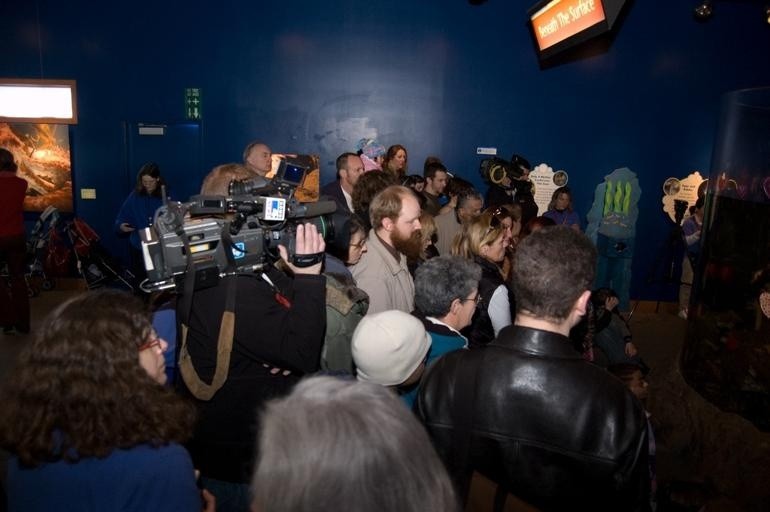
[527,0,620,59]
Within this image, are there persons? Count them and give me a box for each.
[678,198,704,320]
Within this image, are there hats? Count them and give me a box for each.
[357,139,386,159]
[350,309,432,386]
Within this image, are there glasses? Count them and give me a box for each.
[349,239,365,248]
[487,215,500,235]
[493,206,508,216]
[464,294,481,305]
[138,338,161,352]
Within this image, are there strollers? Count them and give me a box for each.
[0,206,60,297]
[48,210,146,305]
